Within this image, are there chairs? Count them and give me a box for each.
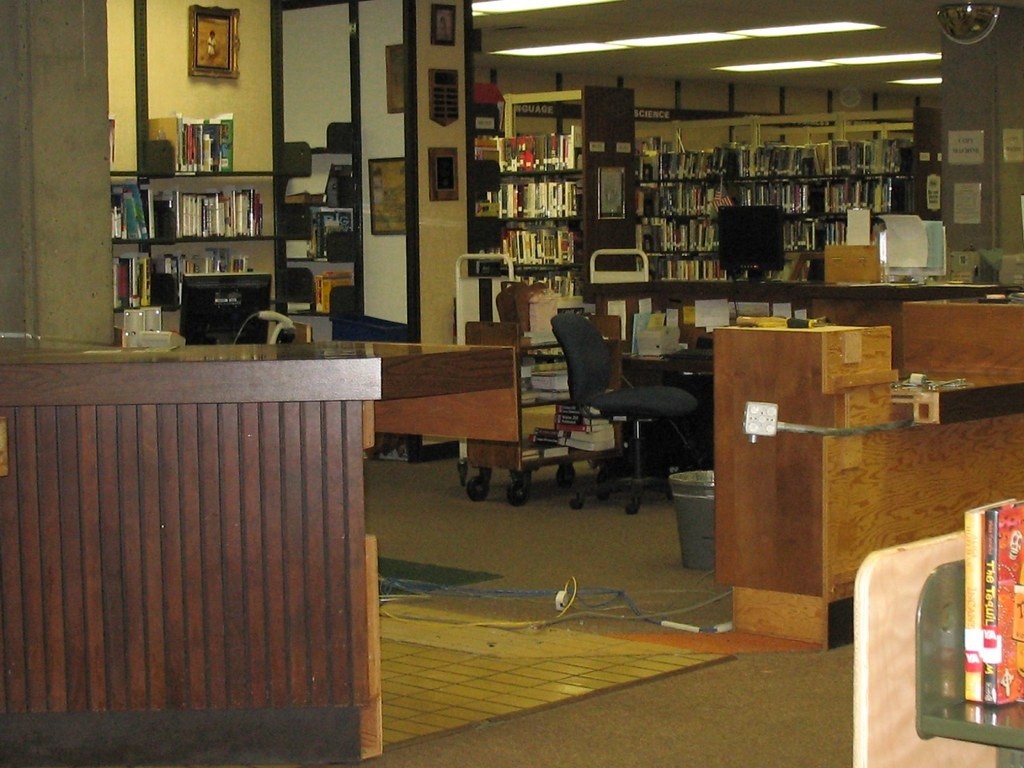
[550,311,702,516]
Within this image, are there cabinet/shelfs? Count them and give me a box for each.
[108,103,944,509]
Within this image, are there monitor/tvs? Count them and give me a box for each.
[179,273,272,344]
[717,205,785,282]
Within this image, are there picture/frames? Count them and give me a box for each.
[429,3,457,46]
[367,156,405,236]
[427,147,459,202]
[186,3,242,81]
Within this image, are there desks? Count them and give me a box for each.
[0,340,526,768]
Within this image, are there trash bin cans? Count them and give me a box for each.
[668,468,716,572]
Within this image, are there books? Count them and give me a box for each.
[502,220,581,300]
[964,499,1024,705]
[468,181,583,216]
[153,188,263,237]
[474,125,583,171]
[636,137,913,280]
[528,401,617,450]
[113,247,254,311]
[146,113,234,174]
[107,184,150,239]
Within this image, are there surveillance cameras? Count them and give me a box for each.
[936,4,1000,45]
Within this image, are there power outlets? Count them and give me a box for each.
[743,398,780,438]
